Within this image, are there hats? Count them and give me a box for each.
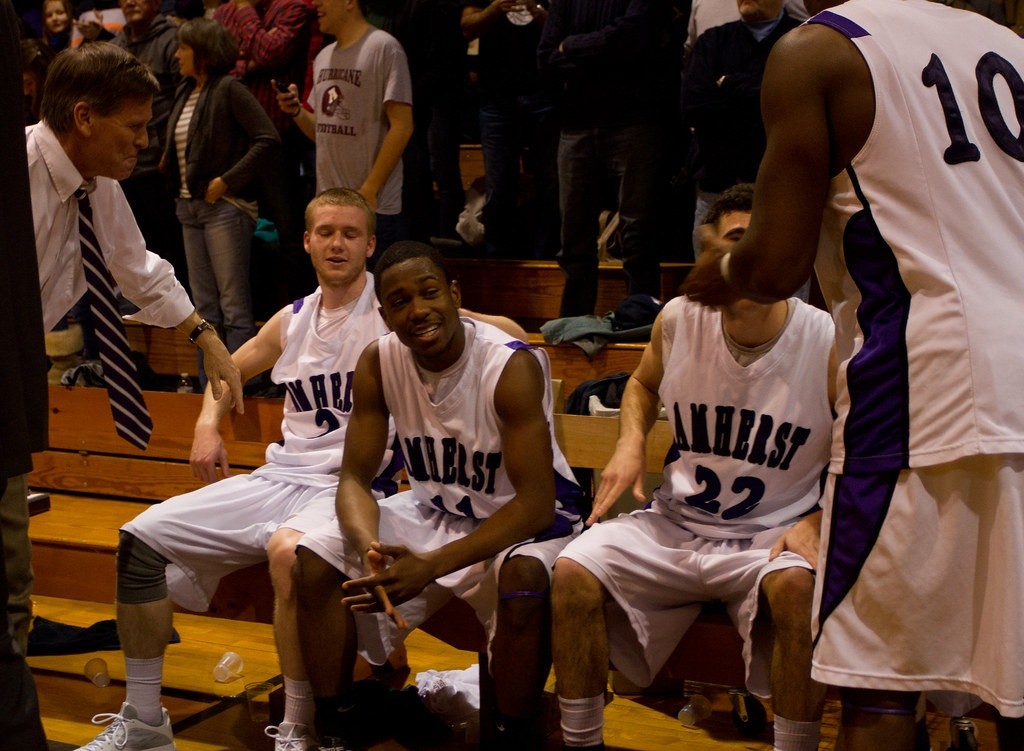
[610,295,664,331]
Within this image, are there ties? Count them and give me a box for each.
[72,185,154,452]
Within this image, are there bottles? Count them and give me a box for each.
[177,372,193,394]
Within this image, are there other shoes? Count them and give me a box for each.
[317,732,352,751]
[115,289,143,323]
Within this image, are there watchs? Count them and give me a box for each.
[188,318,212,344]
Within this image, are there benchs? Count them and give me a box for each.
[27,138,1005,750]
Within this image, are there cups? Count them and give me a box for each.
[84,658,111,689]
[678,694,713,725]
[244,682,275,723]
[213,651,243,682]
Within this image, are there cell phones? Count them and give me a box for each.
[276,82,299,107]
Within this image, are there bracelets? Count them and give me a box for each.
[291,108,300,117]
[720,252,731,283]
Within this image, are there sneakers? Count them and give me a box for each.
[265,719,314,751]
[72,701,175,751]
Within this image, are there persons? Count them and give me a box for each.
[677,0,1024,751]
[0,40,245,654]
[550,182,838,751]
[20,0,811,393]
[73,186,529,751]
[293,240,589,751]
